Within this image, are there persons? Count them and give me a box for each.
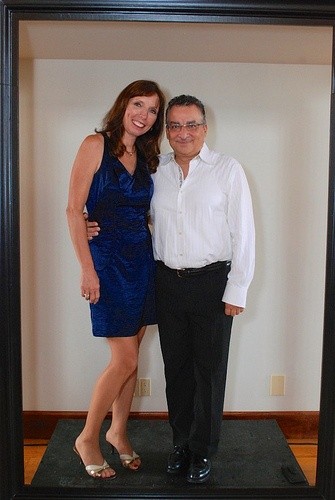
[83,95,256,484]
[66,80,165,479]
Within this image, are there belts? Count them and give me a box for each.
[155,260,227,278]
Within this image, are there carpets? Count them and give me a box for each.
[30,418,309,487]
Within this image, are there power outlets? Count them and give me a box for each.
[133,379,150,396]
[270,375,285,396]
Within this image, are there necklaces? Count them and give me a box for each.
[126,151,135,155]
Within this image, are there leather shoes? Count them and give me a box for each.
[186,455,212,483]
[166,445,190,473]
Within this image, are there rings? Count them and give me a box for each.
[83,294,89,297]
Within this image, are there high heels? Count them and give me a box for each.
[105,437,142,471]
[73,445,117,480]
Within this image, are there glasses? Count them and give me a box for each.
[166,122,204,131]
